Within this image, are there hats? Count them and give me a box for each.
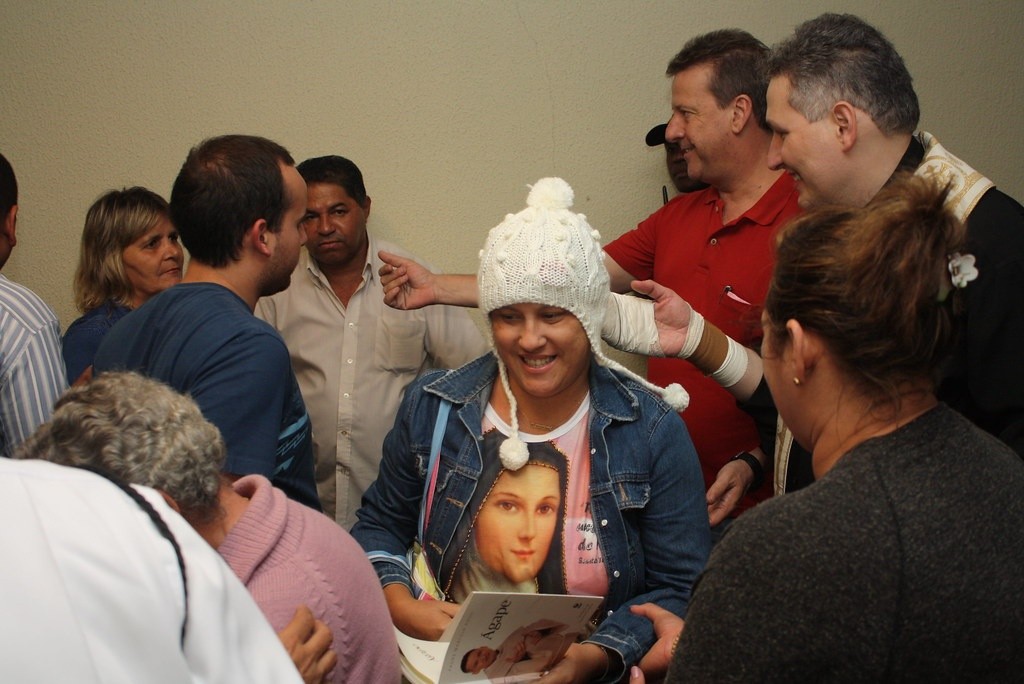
[475,175,691,470]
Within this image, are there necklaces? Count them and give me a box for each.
[515,393,586,432]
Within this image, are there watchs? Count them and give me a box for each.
[729,449,764,493]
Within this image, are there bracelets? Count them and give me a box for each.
[670,631,681,653]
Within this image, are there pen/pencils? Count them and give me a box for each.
[720,285,732,297]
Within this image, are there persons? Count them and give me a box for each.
[644,124,711,194]
[0,455,306,683]
[346,175,711,683]
[629,168,1024,684]
[61,184,184,385]
[376,25,812,543]
[10,370,403,683]
[92,135,319,512]
[461,618,586,678]
[604,12,1024,494]
[254,155,492,532]
[0,154,71,457]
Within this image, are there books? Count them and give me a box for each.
[393,592,604,684]
